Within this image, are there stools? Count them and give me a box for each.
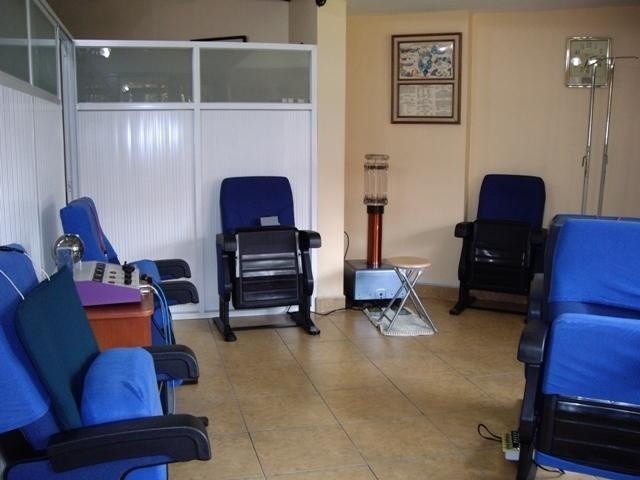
[377,255,439,333]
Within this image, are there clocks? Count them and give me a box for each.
[567,34,614,88]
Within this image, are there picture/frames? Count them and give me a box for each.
[391,32,462,125]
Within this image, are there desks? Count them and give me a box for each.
[84,278,155,348]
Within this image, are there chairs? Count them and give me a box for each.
[516,211,639,479]
[213,175,322,342]
[449,174,548,323]
[0,241,212,479]
[59,199,200,345]
